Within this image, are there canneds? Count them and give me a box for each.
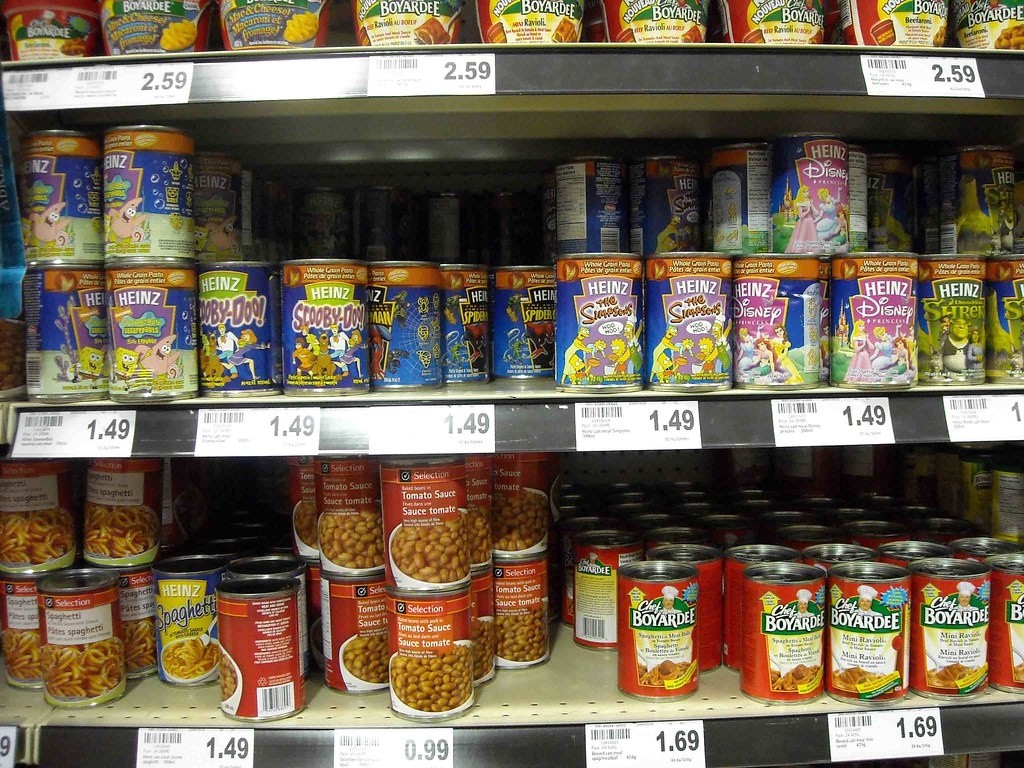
[0,441,1024,723]
[0,125,1024,406]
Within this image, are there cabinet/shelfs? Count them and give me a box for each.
[0,40,1024,768]
